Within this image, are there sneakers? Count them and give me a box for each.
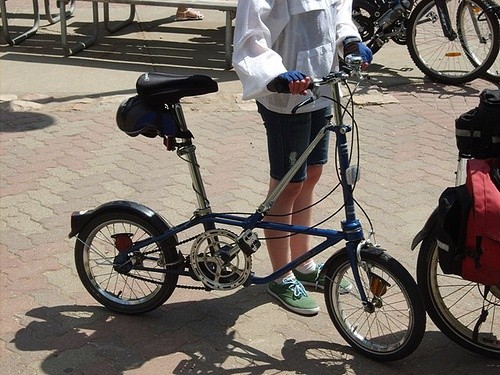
[292,262,352,294]
[267,278,320,315]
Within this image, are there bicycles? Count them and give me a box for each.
[411,88,500,360]
[338,0,500,84]
[68,54,427,362]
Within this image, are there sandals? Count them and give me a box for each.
[176,9,204,20]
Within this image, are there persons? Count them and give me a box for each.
[230,1,375,316]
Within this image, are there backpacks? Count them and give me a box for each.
[436,159,500,286]
